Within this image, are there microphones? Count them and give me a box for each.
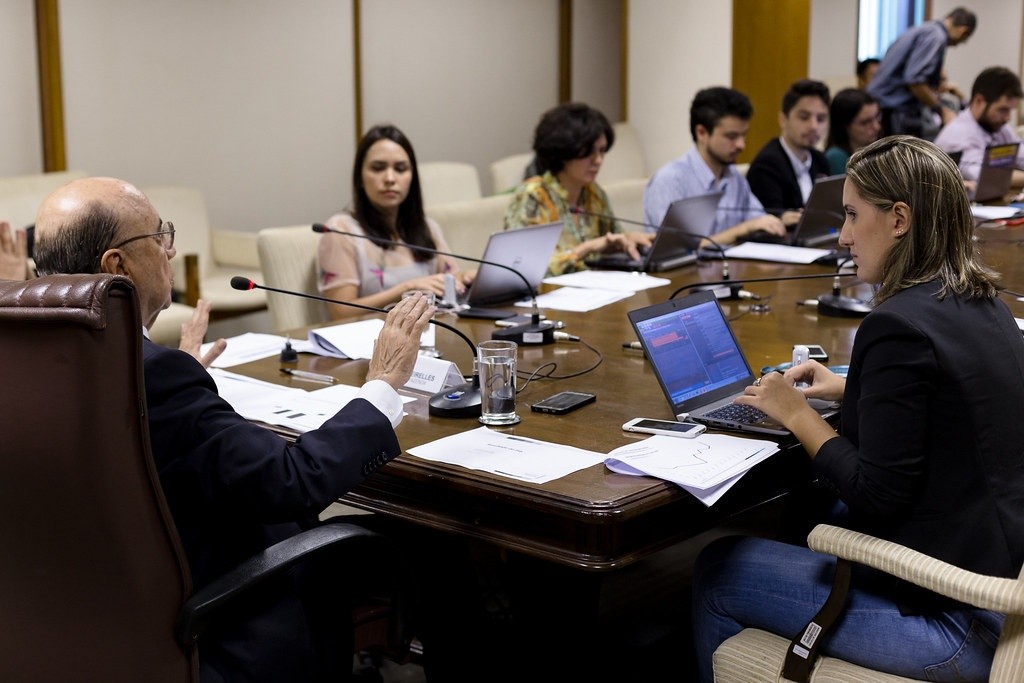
[569,207,744,299]
[231,277,483,418]
[309,222,557,348]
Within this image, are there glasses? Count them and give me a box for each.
[115,221,175,253]
[852,111,882,126]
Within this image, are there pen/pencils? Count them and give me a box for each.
[279,368,339,383]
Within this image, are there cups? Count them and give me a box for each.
[401,289,436,354]
[476,339,517,425]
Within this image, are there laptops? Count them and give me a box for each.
[945,150,962,166]
[966,142,1020,203]
[584,192,723,275]
[627,290,840,440]
[735,175,847,247]
[434,223,565,306]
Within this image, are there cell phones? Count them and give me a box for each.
[622,417,707,439]
[793,344,828,362]
[531,391,596,415]
[456,308,517,321]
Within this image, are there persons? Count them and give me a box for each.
[931,67,1024,190]
[502,102,658,279]
[639,87,803,247]
[690,135,1024,683]
[312,123,476,322]
[33,177,438,683]
[745,80,832,233]
[822,7,977,175]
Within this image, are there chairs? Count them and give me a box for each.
[0,121,1024,682]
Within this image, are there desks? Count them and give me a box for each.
[187,180,1024,682]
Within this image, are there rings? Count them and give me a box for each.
[757,378,761,386]
